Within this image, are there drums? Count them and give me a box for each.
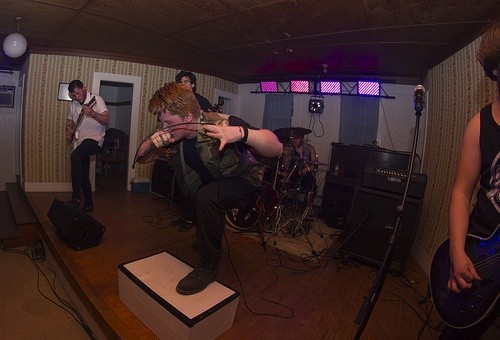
[225,194,263,231]
[279,156,305,172]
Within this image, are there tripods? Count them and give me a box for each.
[261,135,323,257]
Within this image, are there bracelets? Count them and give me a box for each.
[238,126,242,139]
[240,125,249,143]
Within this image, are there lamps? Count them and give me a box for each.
[308,93,324,114]
[3,15,27,58]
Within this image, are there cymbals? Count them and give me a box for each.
[273,127,312,137]
[307,161,327,165]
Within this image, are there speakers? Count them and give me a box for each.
[318,141,428,275]
[48,198,107,250]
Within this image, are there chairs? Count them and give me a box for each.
[96,126,127,173]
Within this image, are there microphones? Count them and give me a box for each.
[413,84,425,112]
[244,211,253,221]
[137,132,171,162]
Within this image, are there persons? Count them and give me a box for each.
[137,81,283,296]
[65,80,110,212]
[169,72,212,232]
[448,22,500,340]
[292,134,318,218]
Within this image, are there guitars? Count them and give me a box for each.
[69,96,97,153]
[208,99,226,113]
[429,223,500,331]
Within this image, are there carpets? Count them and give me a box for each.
[225,212,342,262]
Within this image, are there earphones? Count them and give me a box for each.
[187,115,191,119]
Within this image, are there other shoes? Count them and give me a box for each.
[67,198,93,212]
[175,264,218,295]
[172,217,193,231]
